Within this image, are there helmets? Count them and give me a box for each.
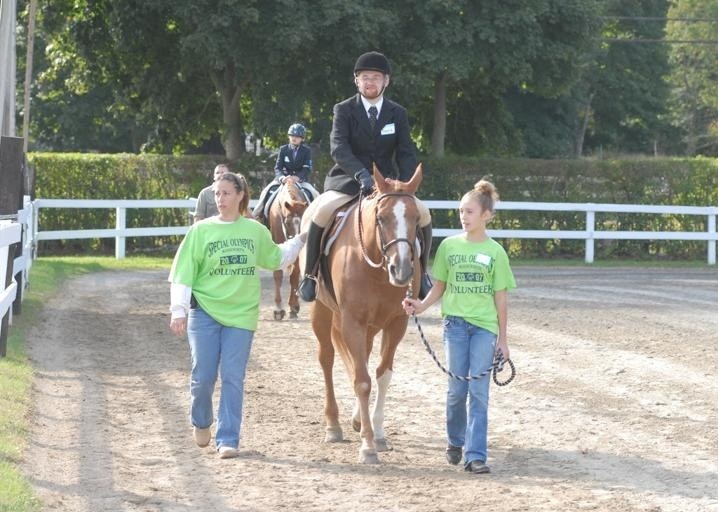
[287,123,306,139]
[353,51,391,76]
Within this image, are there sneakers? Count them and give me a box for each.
[193,424,212,447]
[217,446,238,459]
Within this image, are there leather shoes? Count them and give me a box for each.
[469,460,489,473]
[444,442,464,466]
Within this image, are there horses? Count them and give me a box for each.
[310,163,424,468]
[267,177,309,322]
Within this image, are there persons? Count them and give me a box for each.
[401,180,517,474]
[297,51,433,303]
[168,172,311,459]
[259,124,319,230]
[193,163,230,223]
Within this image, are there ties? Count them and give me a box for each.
[368,106,378,128]
[293,149,297,160]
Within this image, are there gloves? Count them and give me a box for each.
[354,168,375,196]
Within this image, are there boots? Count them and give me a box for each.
[417,220,433,299]
[297,220,325,302]
